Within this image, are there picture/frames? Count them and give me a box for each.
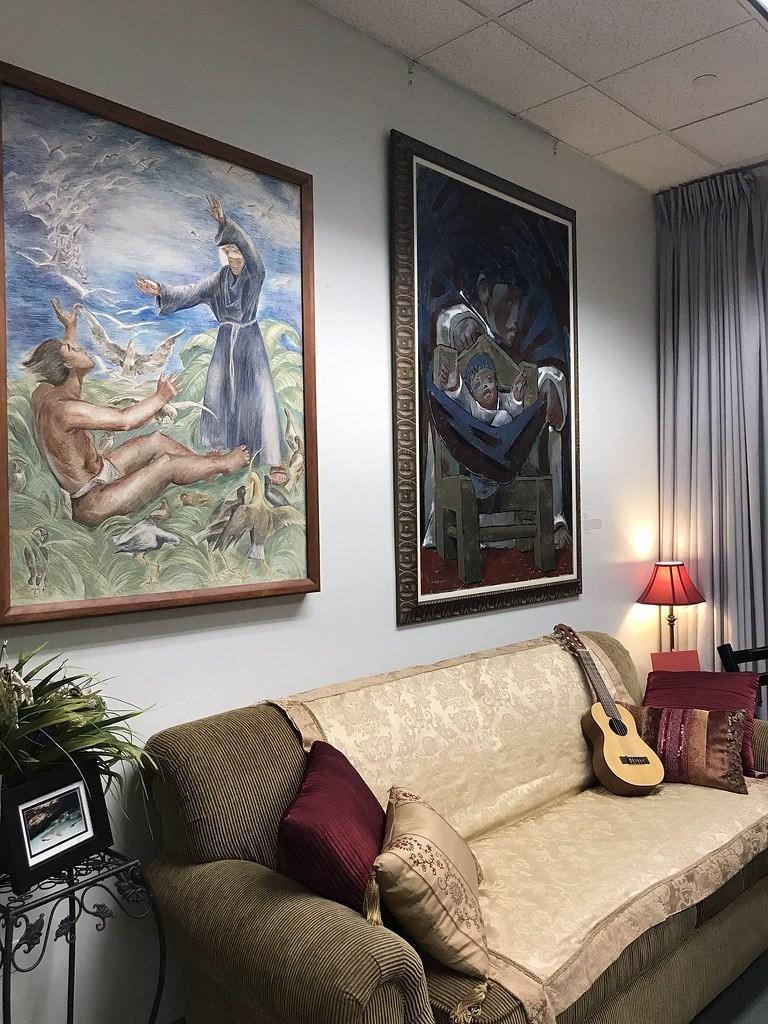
[2,757,115,896]
[390,129,584,630]
[0,60,321,627]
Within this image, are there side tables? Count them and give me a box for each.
[0,847,165,1024]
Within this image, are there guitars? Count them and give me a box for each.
[552,623,667,798]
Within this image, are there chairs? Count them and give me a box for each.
[717,642,768,686]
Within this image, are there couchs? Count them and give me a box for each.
[135,630,768,1024]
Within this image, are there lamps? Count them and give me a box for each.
[636,561,706,651]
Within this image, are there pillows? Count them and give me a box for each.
[612,700,749,796]
[278,740,385,916]
[362,785,489,1023]
[641,671,768,779]
